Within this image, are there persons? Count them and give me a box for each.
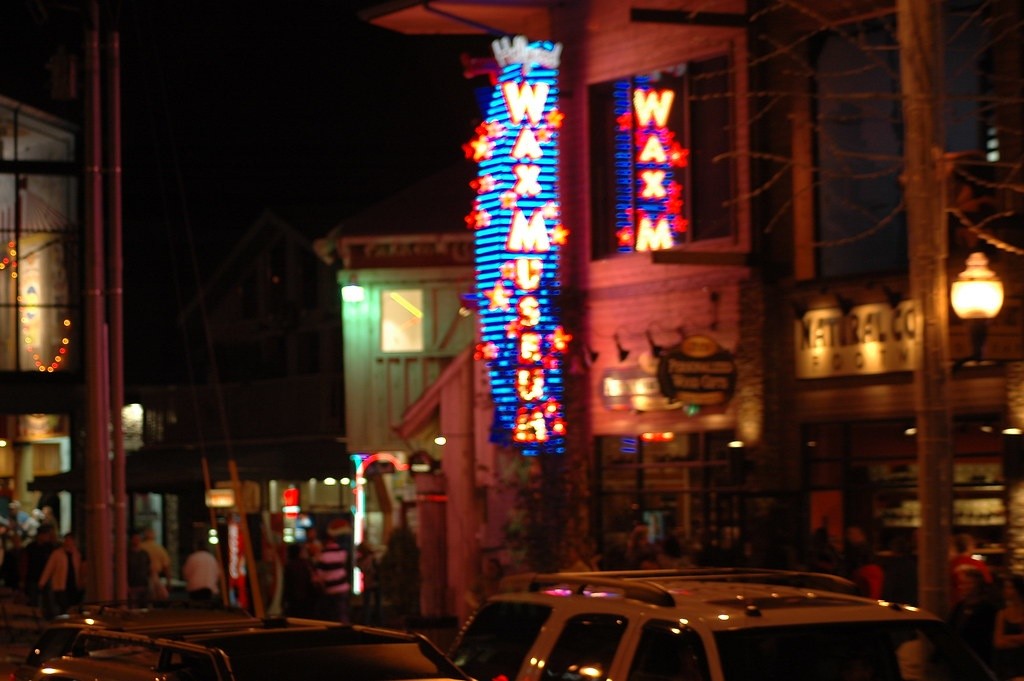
[19,524,350,624]
[476,558,503,600]
[539,526,1024,681]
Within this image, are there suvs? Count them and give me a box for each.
[12,603,480,681]
[447,564,1004,681]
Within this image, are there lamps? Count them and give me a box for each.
[645,320,679,357]
[612,325,637,361]
[951,240,1005,364]
[820,283,854,315]
[866,280,902,308]
[580,325,610,364]
[782,290,808,319]
